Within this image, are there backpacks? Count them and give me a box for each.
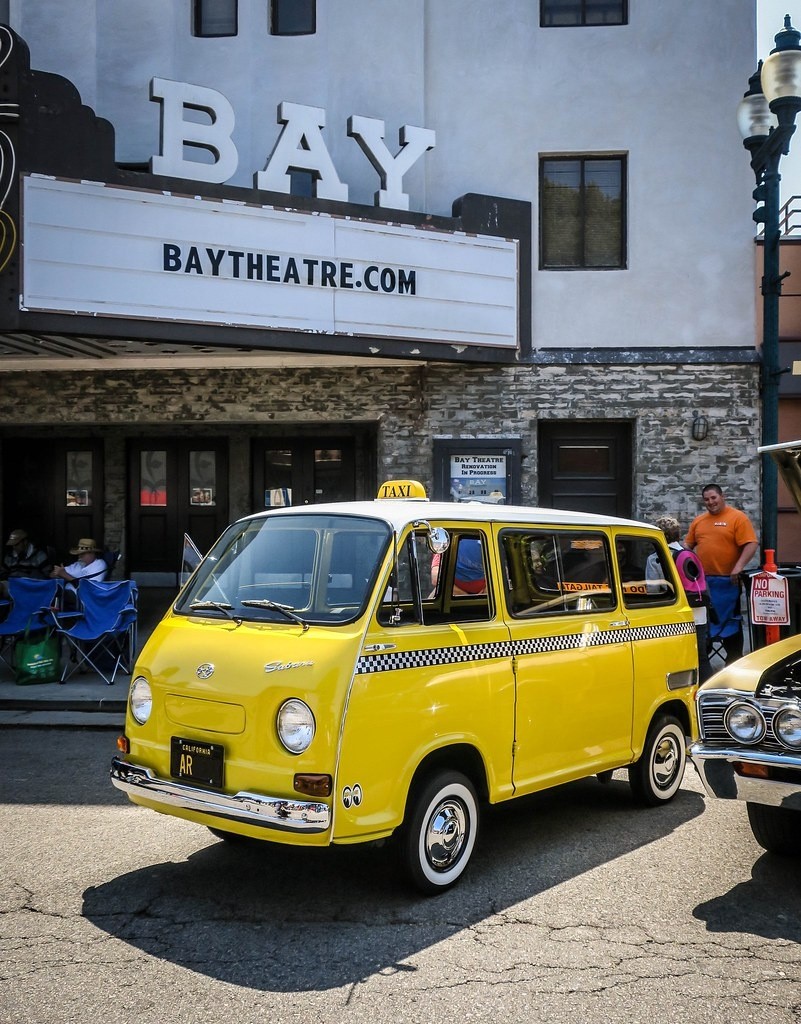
[658,544,711,608]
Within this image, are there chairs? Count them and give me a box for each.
[0,549,138,685]
[703,575,743,664]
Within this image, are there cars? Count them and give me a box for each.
[687,632,801,851]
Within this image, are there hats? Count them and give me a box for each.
[70,538,100,555]
[6,529,29,545]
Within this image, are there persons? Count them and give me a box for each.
[642,515,713,686]
[683,484,761,668]
[0,529,53,599]
[49,538,109,613]
[421,536,488,598]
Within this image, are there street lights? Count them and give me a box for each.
[733,12,801,657]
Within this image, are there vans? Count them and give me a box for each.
[108,480,701,898]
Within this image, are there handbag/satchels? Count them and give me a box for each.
[11,610,62,686]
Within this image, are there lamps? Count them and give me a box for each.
[692,417,708,441]
